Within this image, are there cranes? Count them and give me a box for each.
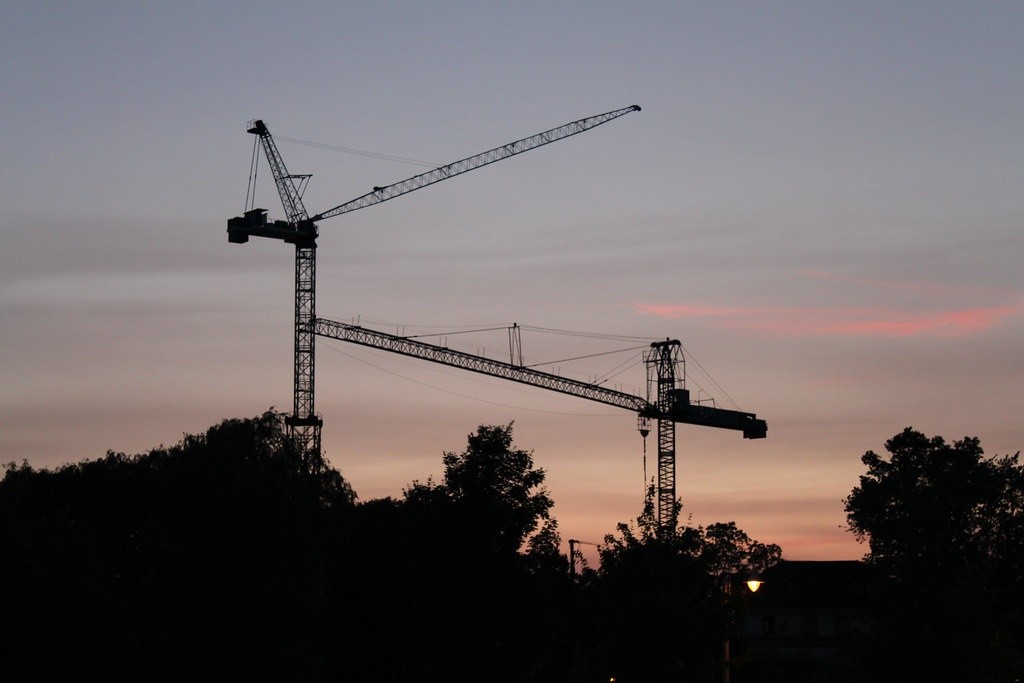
[226,103,643,472]
[315,316,771,548]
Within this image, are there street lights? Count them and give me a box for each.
[722,551,766,683]
